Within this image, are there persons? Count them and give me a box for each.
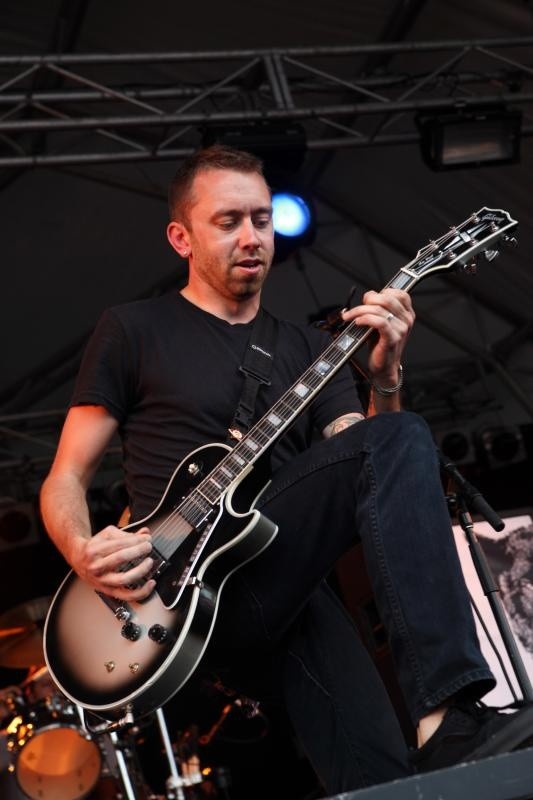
[39,141,533,786]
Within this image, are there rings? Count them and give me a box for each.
[386,313,394,322]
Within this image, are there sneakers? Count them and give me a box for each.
[415,694,533,772]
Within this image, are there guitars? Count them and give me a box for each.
[43,202,521,715]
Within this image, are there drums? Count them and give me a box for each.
[8,702,102,800]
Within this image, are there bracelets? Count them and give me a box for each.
[375,363,403,392]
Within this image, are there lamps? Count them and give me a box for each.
[265,177,321,263]
[413,101,526,174]
